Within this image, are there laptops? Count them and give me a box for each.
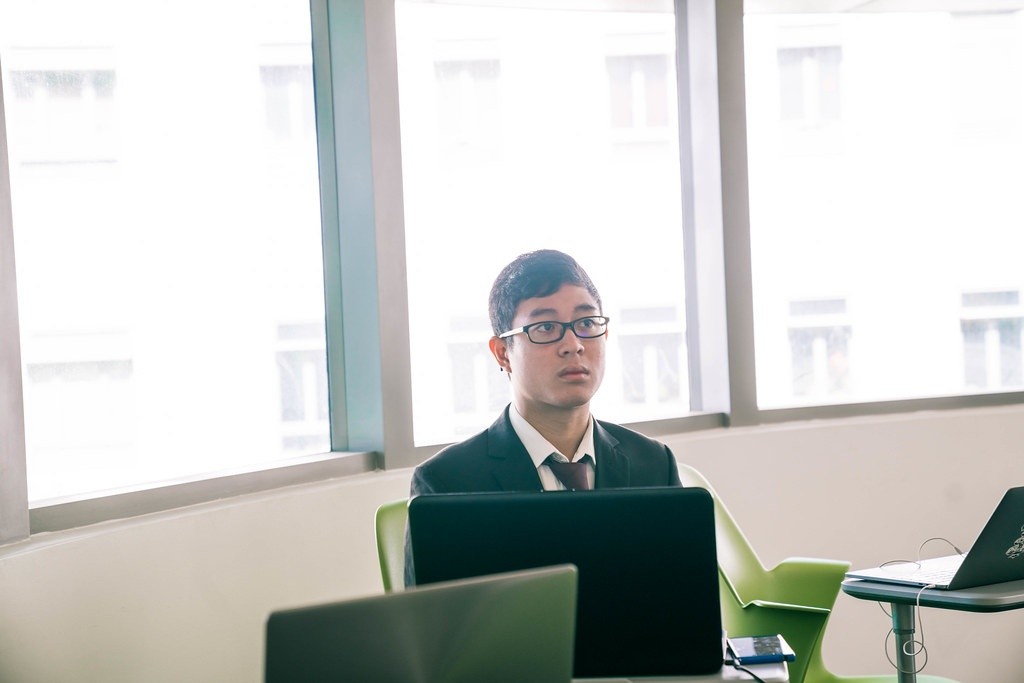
[264,562,578,683]
[846,486,1024,590]
[404,485,721,676]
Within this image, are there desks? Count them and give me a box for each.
[841,578,1024,683]
[575,663,789,683]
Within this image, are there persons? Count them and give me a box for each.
[402,249,683,592]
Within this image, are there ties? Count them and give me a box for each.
[544,454,591,489]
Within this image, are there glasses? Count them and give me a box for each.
[498,316,610,371]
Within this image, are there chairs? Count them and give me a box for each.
[674,462,962,683]
[375,497,414,593]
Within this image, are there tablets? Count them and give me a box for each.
[726,634,795,666]
